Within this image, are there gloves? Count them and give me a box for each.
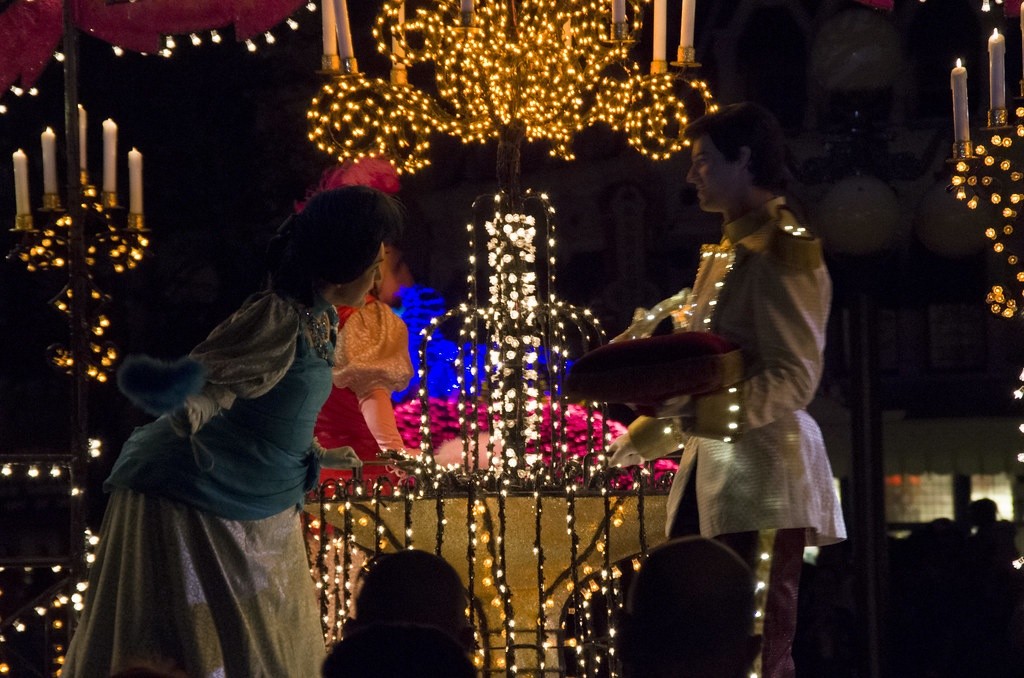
[635,395,696,420]
[169,399,202,440]
[402,446,421,460]
[608,433,646,469]
[313,436,362,469]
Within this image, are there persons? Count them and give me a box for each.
[319,550,479,678]
[614,536,763,678]
[292,159,413,499]
[59,184,404,678]
[370,233,412,308]
[608,100,847,678]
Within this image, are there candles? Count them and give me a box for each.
[680,0,696,48]
[987,27,1006,109]
[461,0,474,12]
[128,147,143,214]
[77,104,87,170]
[322,0,355,58]
[612,0,626,23]
[950,57,970,142]
[391,2,406,69]
[102,118,117,193]
[41,126,58,194]
[13,149,31,214]
[653,0,666,61]
